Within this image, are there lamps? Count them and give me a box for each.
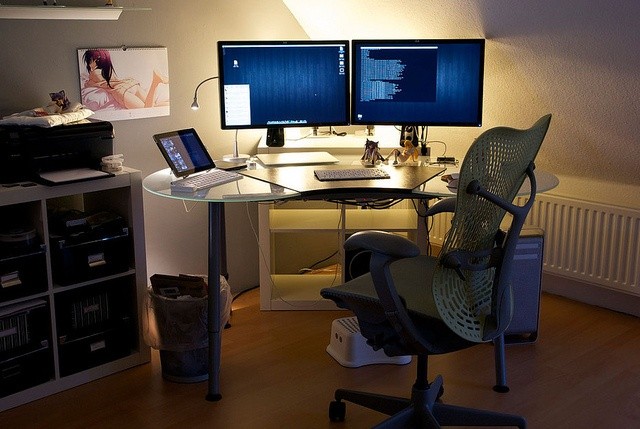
[189,76,253,162]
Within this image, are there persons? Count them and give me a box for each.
[32,90,71,117]
[358,136,419,167]
[80,48,169,111]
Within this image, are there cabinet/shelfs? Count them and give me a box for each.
[0,166,153,416]
[256,134,431,311]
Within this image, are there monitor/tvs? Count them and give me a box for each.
[152,128,215,179]
[217,40,351,130]
[351,38,486,127]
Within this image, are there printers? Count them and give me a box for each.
[0,116,114,187]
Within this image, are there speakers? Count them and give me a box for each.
[399,126,419,148]
[266,128,284,146]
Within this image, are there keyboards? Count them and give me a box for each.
[314,168,390,181]
[170,169,243,193]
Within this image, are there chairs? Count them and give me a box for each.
[319,113,553,429]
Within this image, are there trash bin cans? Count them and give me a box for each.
[148,274,227,383]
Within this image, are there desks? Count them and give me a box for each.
[142,152,560,402]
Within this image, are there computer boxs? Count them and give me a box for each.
[493,212,545,345]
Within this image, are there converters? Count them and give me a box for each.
[246,160,256,169]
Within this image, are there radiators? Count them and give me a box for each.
[426,189,640,299]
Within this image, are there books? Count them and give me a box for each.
[1,278,21,289]
[58,210,86,228]
[0,270,19,283]
